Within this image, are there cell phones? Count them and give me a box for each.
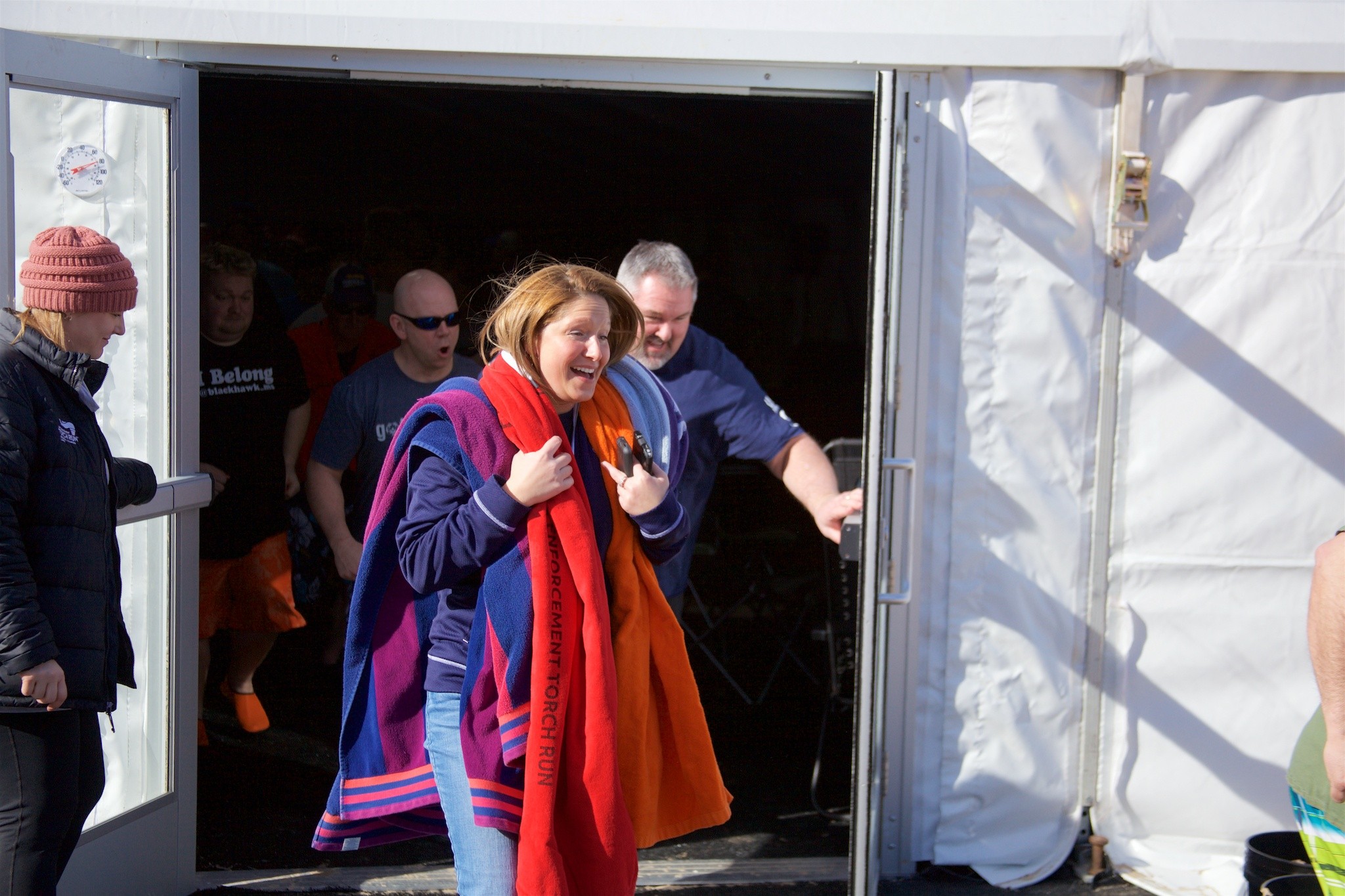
[632,430,654,476]
[615,436,634,477]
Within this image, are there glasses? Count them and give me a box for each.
[393,310,463,331]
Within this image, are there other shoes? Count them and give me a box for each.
[197,716,210,747]
[220,676,270,733]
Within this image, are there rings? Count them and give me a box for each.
[846,495,852,500]
[619,477,627,488]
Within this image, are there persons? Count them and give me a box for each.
[616,243,862,621]
[1286,526,1345,896]
[0,225,157,896]
[304,269,483,578]
[196,241,312,748]
[312,262,733,896]
[287,264,399,667]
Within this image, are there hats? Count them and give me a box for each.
[19,225,138,313]
